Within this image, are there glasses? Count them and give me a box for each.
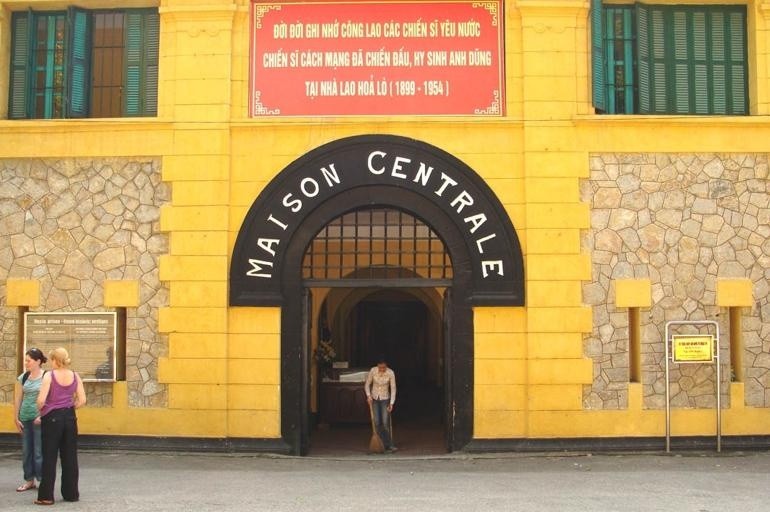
[31,349,37,353]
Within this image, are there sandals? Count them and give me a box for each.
[16,480,36,492]
[34,499,54,504]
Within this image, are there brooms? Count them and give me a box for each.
[368,397,385,454]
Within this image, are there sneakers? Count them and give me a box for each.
[389,445,397,451]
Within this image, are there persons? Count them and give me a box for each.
[14,347,87,506]
[363,357,397,453]
